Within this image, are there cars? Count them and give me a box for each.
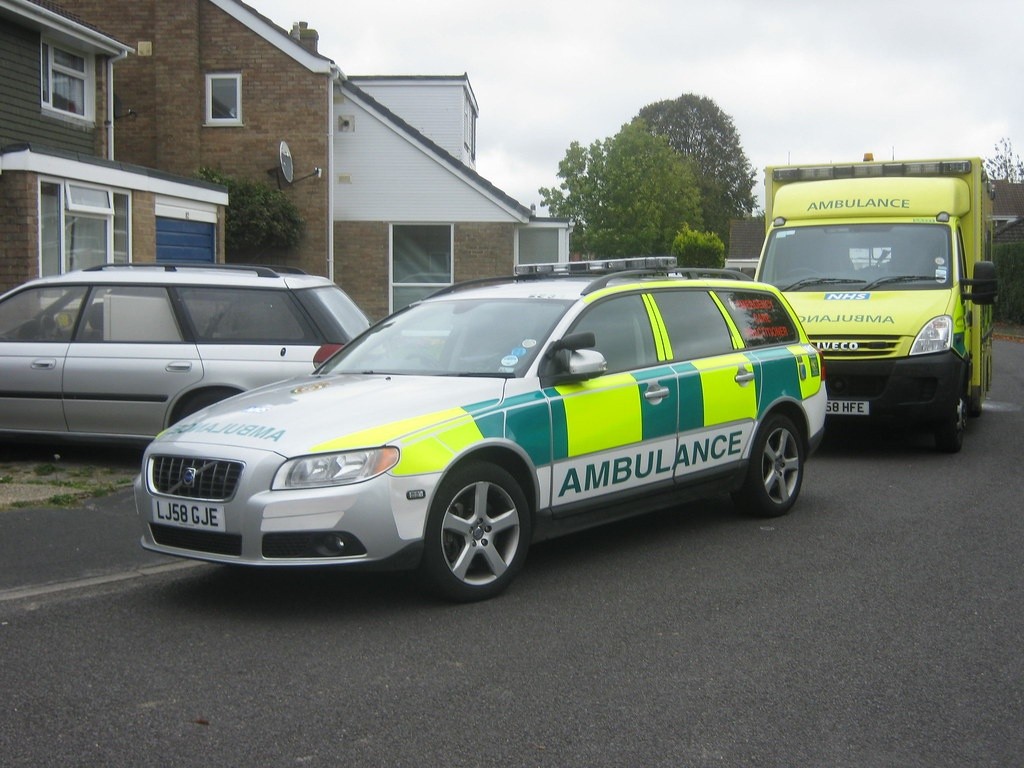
[0,260,372,454]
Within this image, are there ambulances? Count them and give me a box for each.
[750,152,996,454]
[130,255,829,601]
